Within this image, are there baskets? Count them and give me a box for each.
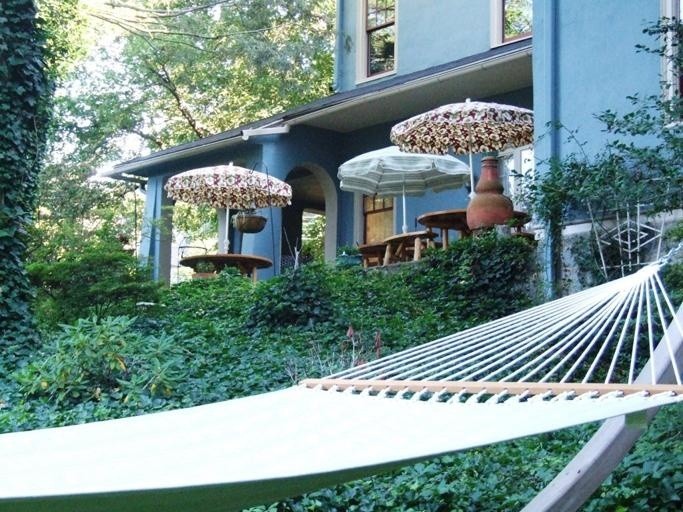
[234,214,268,234]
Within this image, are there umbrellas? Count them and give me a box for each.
[389,96,533,201]
[163,159,292,255]
[335,145,469,234]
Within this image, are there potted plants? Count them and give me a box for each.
[231,208,268,233]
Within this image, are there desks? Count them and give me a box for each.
[180,254,273,283]
[358,209,531,269]
